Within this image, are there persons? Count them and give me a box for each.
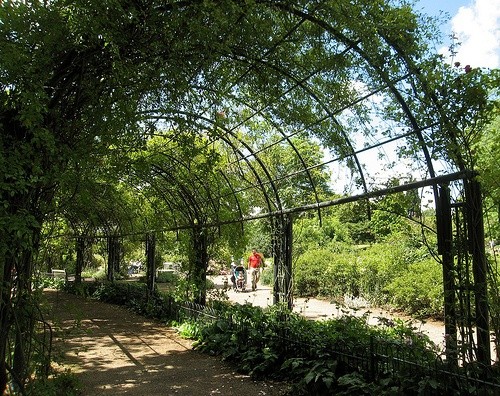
[238,272,245,290]
[248,248,266,291]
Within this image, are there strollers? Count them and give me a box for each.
[230,266,248,293]
